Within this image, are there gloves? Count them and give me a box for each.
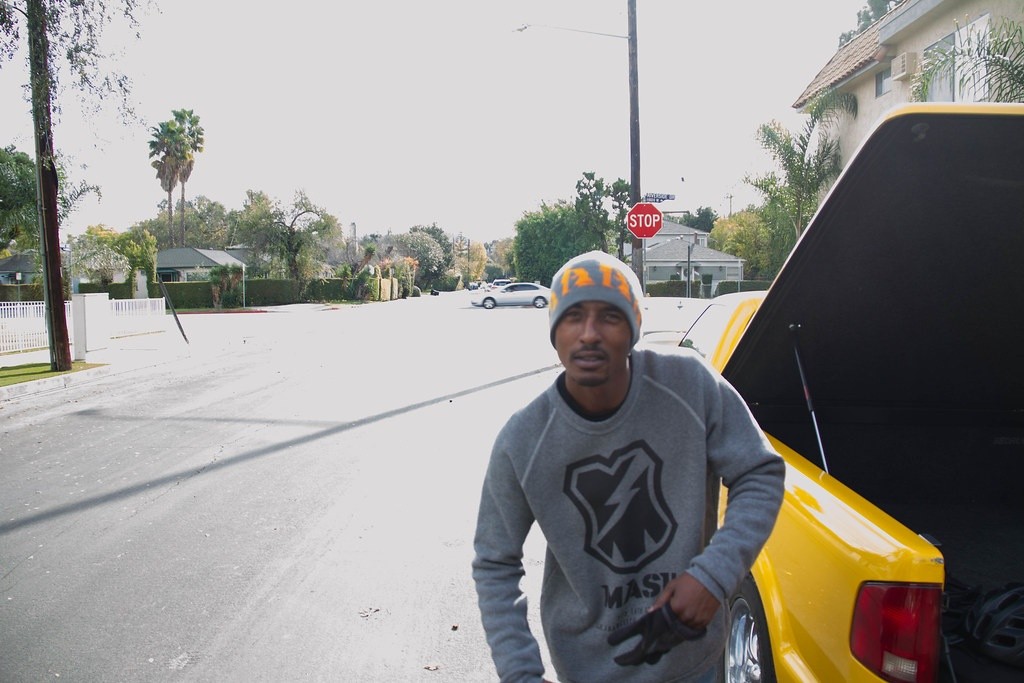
[608,601,708,667]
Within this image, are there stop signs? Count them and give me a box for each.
[627,203,663,239]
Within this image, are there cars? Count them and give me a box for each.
[472,282,551,309]
[469,279,510,293]
[678,102,1024,683]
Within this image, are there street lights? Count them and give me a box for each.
[16,273,21,304]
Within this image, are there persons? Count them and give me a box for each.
[472,249,787,682]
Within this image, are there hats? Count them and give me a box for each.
[548,249,645,351]
